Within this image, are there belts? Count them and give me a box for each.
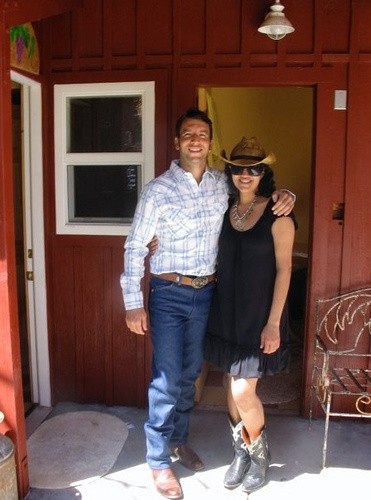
[152,272,217,290]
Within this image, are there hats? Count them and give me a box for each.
[220,136,277,167]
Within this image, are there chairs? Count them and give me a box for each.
[309,288,371,470]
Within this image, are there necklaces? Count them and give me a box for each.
[232,196,257,231]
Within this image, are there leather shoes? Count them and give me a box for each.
[169,444,206,472]
[151,467,183,500]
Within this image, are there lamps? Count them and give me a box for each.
[256,0,296,41]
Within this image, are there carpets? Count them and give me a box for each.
[26,411,128,489]
[222,373,304,405]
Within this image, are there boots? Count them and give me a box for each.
[241,425,271,493]
[223,411,252,489]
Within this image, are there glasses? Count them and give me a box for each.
[228,165,266,176]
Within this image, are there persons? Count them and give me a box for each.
[120,108,296,500]
[147,137,299,492]
